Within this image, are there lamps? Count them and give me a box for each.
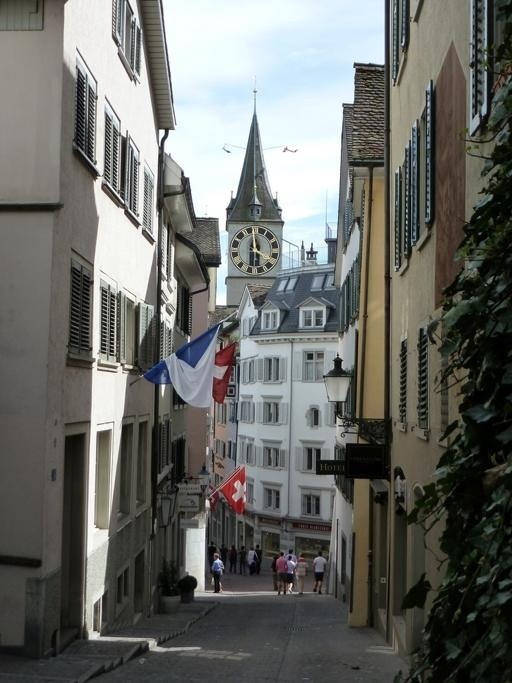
[322,350,393,448]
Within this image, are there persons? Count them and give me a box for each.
[206,541,331,597]
[210,553,225,593]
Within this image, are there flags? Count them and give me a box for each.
[213,340,238,404]
[142,321,222,408]
[208,465,247,515]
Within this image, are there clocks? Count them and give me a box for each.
[229,226,281,276]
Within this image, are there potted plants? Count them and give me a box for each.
[158,557,180,612]
[180,574,197,602]
